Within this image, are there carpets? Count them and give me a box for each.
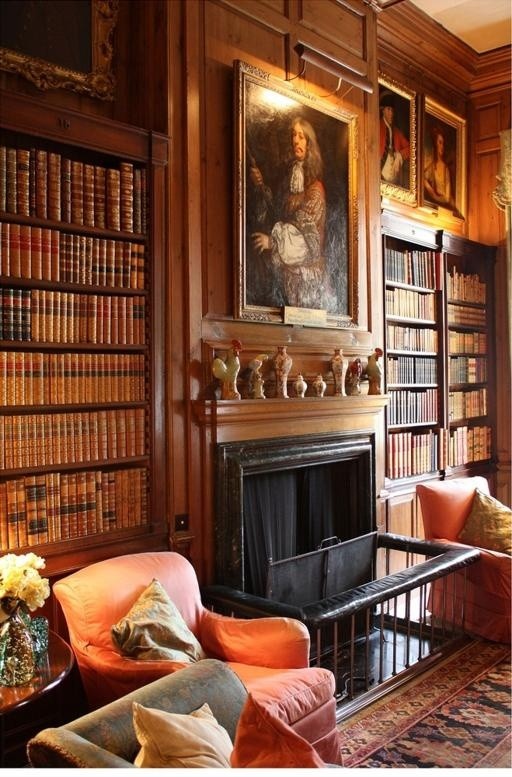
[337,638,512,768]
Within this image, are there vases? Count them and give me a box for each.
[23,616,48,658]
[1,612,36,686]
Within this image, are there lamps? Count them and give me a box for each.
[282,44,375,98]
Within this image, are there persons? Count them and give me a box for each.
[250,118,330,310]
[426,133,450,204]
[376,98,414,182]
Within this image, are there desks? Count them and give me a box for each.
[0,625,73,764]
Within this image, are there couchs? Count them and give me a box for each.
[52,552,343,767]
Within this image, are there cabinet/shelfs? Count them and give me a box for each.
[381,210,497,577]
[1,91,168,582]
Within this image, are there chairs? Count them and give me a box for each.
[26,659,253,768]
[415,475,512,643]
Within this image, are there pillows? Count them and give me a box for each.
[231,692,346,767]
[458,487,512,556]
[131,702,235,767]
[110,578,207,664]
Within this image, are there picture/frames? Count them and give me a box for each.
[0,0,118,104]
[377,70,419,209]
[418,91,468,226]
[230,59,364,329]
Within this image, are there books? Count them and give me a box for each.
[450,427,494,465]
[0,144,149,231]
[0,353,147,411]
[445,358,490,387]
[0,293,150,348]
[383,287,439,322]
[449,304,488,328]
[448,390,487,420]
[386,247,436,290]
[447,266,488,304]
[386,323,439,357]
[0,222,149,289]
[0,415,147,472]
[390,390,437,426]
[447,330,488,355]
[389,358,437,386]
[0,467,156,552]
[386,431,448,479]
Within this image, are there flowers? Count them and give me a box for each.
[0,552,51,618]
